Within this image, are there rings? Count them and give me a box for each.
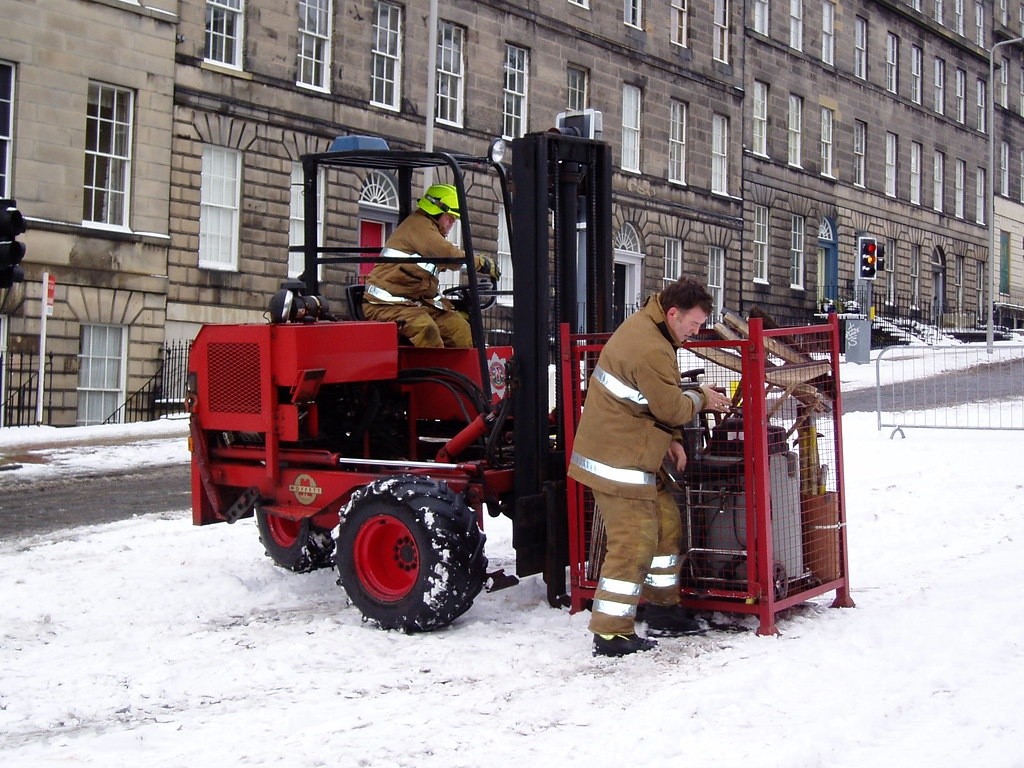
[715,403,717,408]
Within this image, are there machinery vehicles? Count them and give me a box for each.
[185,126,746,636]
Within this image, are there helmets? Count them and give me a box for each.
[416,184,460,216]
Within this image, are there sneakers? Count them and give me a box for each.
[591,633,658,658]
[648,613,710,636]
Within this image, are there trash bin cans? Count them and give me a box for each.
[844,318,874,364]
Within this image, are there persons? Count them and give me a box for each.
[566,276,733,658]
[357,183,500,350]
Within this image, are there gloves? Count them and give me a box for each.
[476,254,501,280]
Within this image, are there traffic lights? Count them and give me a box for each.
[0,199,27,288]
[858,236,877,280]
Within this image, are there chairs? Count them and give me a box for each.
[345,283,364,322]
